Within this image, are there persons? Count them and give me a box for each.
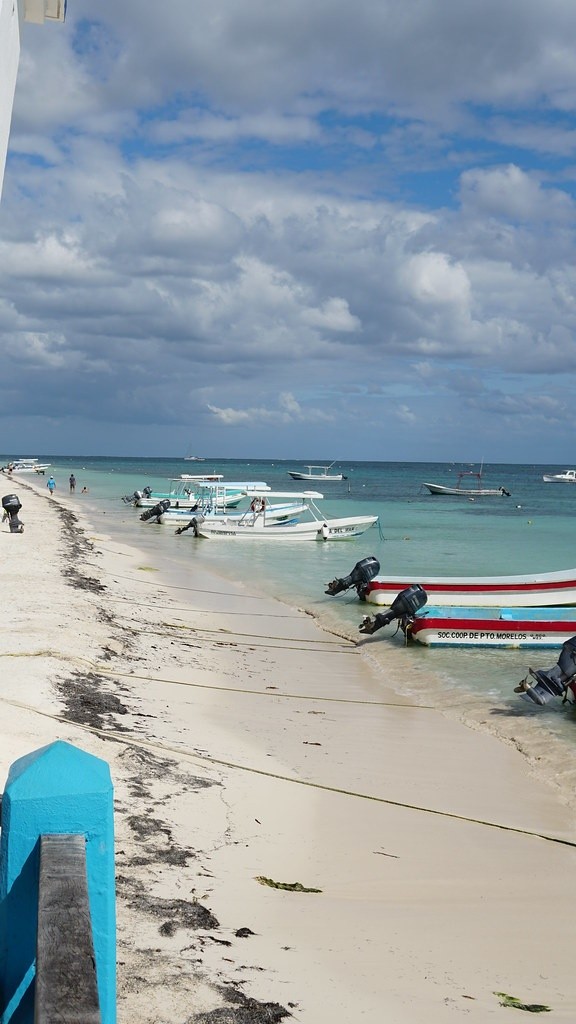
[47,476,56,497]
[69,473,76,494]
[8,464,13,475]
[81,487,88,494]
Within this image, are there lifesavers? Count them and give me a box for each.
[249,498,266,513]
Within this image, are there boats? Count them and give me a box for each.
[543,469,576,483]
[3,461,48,473]
[287,465,348,482]
[174,490,377,542]
[185,456,205,461]
[123,474,245,509]
[322,555,576,606]
[357,584,576,650]
[19,458,52,467]
[140,481,308,528]
[423,483,512,497]
